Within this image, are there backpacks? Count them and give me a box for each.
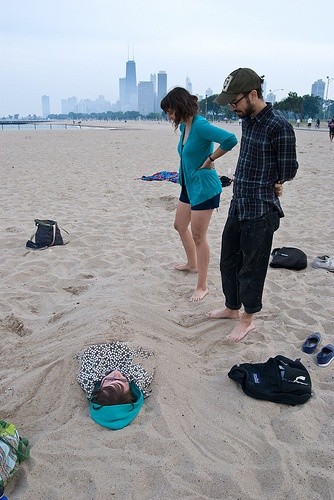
[228,355,312,406]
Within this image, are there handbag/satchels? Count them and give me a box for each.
[25,219,70,251]
[0,419,30,490]
[270,246,308,270]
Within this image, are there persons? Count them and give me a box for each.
[317,119,320,129]
[328,118,334,140]
[297,118,301,127]
[91,369,137,405]
[205,68,299,342]
[308,117,312,126]
[161,87,238,302]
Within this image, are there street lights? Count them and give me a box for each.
[326,76,330,122]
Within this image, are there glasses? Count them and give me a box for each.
[101,377,129,387]
[227,91,252,108]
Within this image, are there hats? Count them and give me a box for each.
[213,68,261,106]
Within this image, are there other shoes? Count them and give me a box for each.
[316,344,334,366]
[303,332,321,353]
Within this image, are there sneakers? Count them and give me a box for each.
[312,255,334,272]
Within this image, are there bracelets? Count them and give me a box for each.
[209,154,214,161]
